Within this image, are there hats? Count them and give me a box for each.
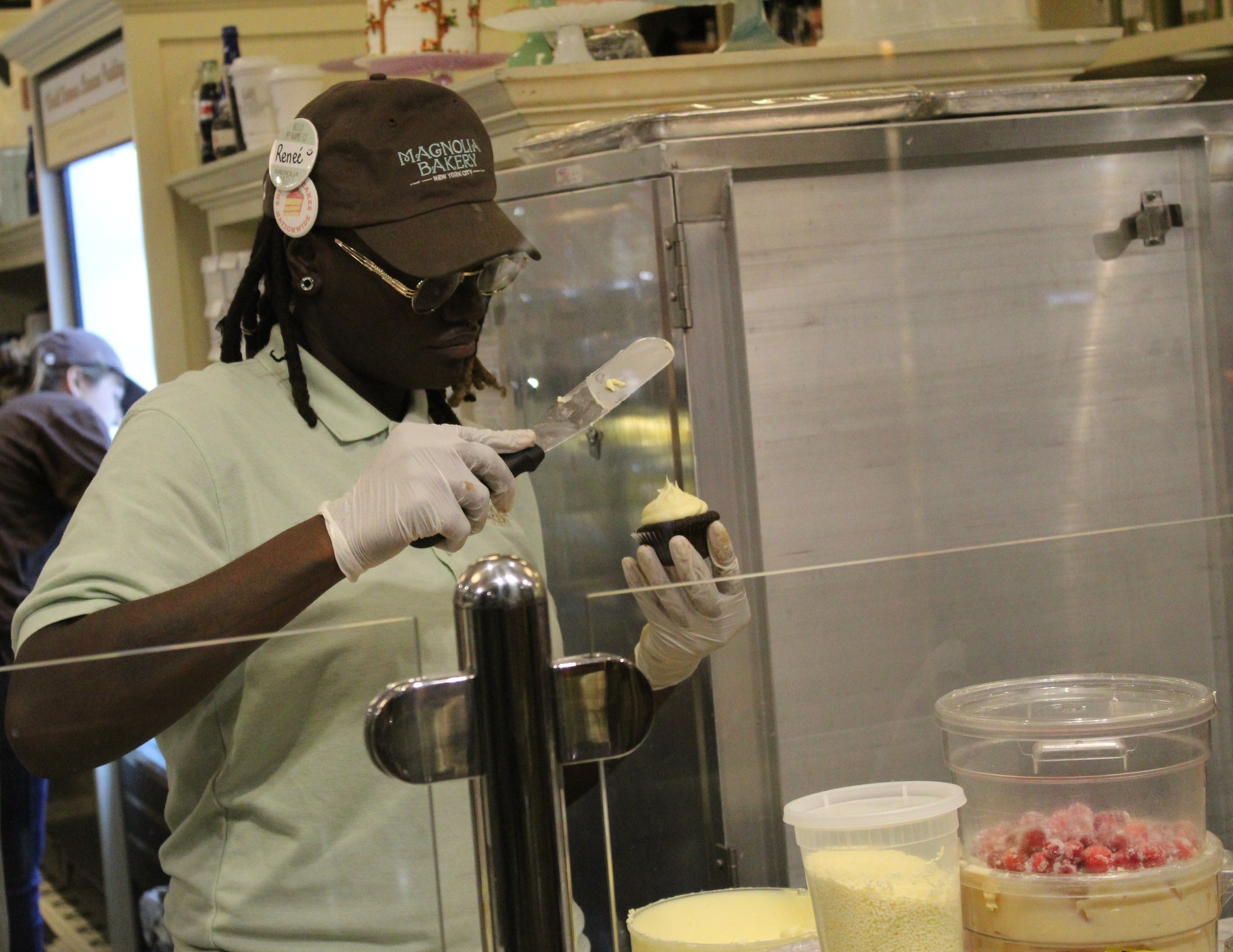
[262,73,541,278]
[29,329,149,415]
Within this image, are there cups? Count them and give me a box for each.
[268,62,323,133]
[227,54,278,147]
[782,781,967,952]
[935,671,1233,951]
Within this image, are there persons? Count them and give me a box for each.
[5,73,751,952]
[0,330,145,952]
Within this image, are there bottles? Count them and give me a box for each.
[26,126,40,213]
[198,27,245,160]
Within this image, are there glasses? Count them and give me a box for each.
[333,236,529,316]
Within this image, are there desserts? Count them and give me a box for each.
[630,474,721,565]
